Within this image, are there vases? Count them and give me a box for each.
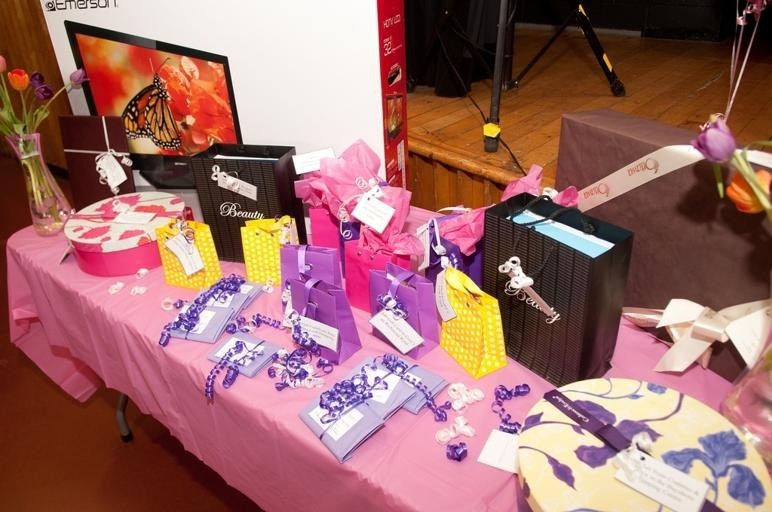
[0,128,77,236]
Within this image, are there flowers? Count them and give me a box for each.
[681,112,772,238]
[0,55,94,221]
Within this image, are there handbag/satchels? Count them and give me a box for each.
[477,190,638,390]
[239,211,301,286]
[153,218,223,291]
[308,171,507,380]
[190,140,305,266]
[279,243,363,367]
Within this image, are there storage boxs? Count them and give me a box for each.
[55,186,190,280]
[512,372,771,512]
[550,104,772,389]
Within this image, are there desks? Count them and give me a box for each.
[3,168,771,512]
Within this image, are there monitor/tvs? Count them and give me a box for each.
[64,19,243,189]
